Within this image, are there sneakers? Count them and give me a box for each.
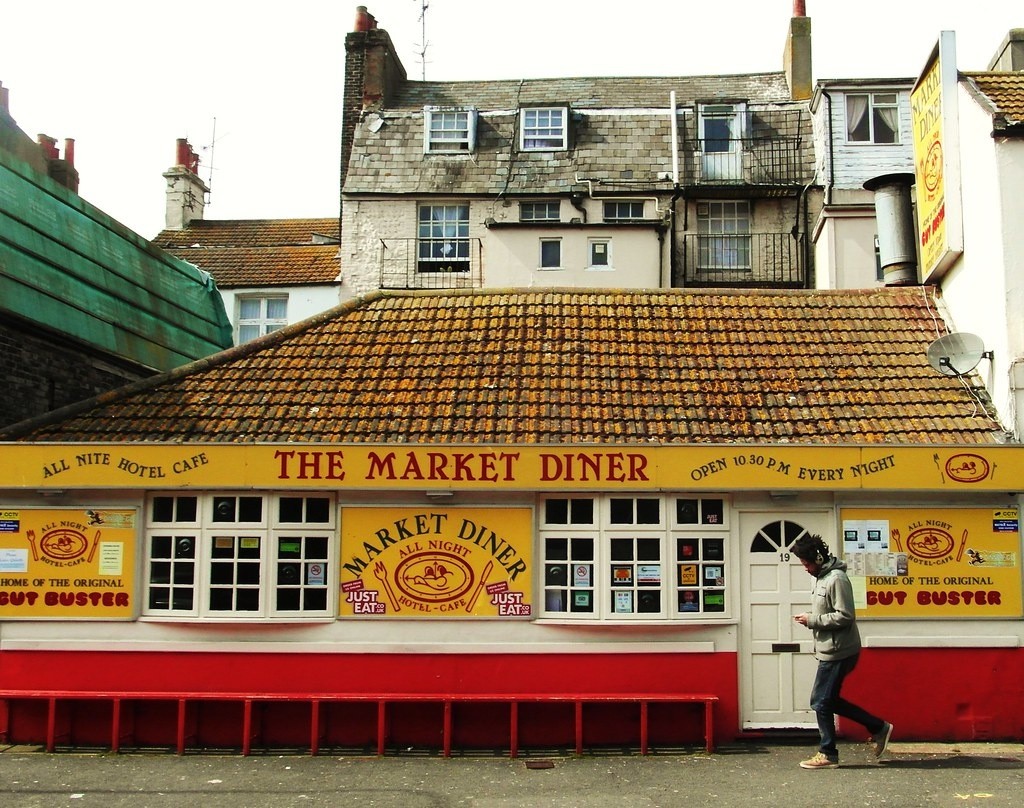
[873,720,893,760]
[799,752,839,769]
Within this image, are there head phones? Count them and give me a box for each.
[814,550,824,565]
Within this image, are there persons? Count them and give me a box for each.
[793,534,893,769]
